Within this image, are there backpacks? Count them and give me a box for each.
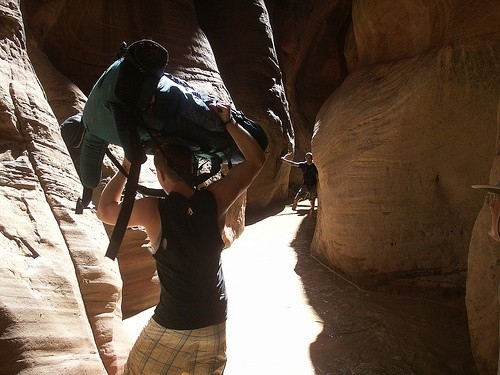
[61,40,268,259]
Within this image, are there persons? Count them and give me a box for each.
[96,102,267,375]
[278,152,320,214]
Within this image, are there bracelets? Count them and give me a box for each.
[224,116,233,125]
